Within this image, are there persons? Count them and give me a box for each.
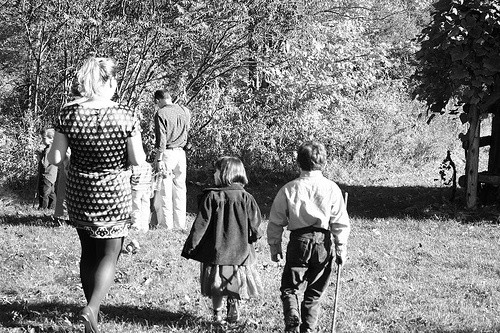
[180,156,264,321]
[129,144,155,231]
[151,89,190,228]
[267,141,350,333]
[35,129,56,209]
[47,58,145,333]
[44,165,70,225]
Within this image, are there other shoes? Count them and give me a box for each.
[226,297,238,321]
[80,306,99,333]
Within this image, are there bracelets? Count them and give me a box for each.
[157,159,161,162]
